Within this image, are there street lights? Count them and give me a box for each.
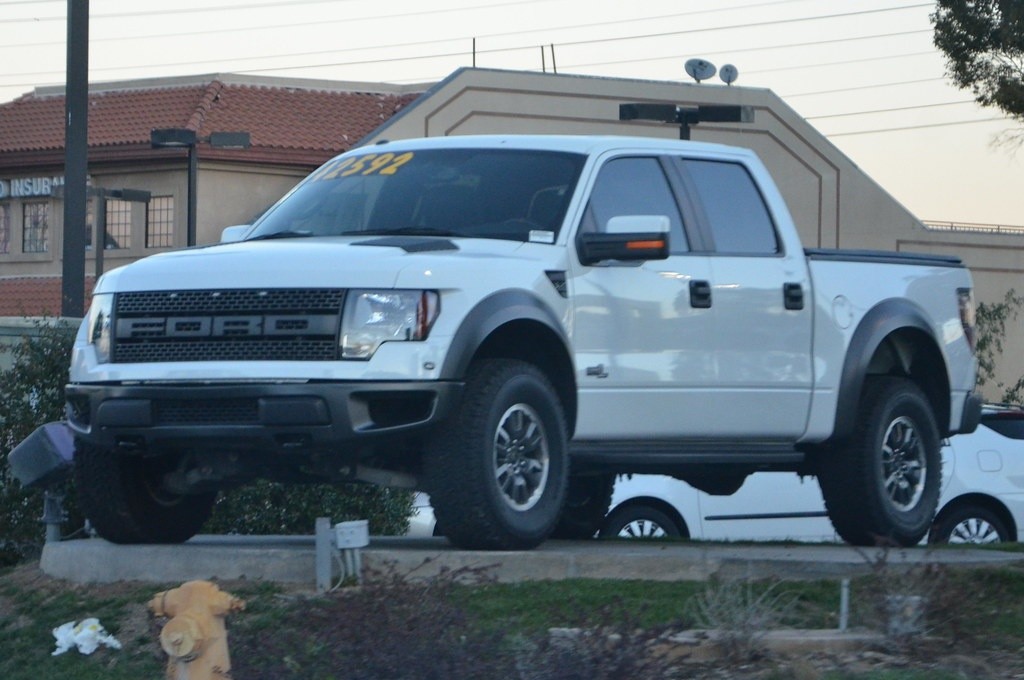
[151,128,250,246]
[52,185,151,284]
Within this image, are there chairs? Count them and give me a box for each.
[527,186,571,221]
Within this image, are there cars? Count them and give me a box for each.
[593,399,1024,544]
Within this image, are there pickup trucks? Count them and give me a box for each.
[64,133,983,549]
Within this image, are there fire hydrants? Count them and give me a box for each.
[146,579,245,680]
